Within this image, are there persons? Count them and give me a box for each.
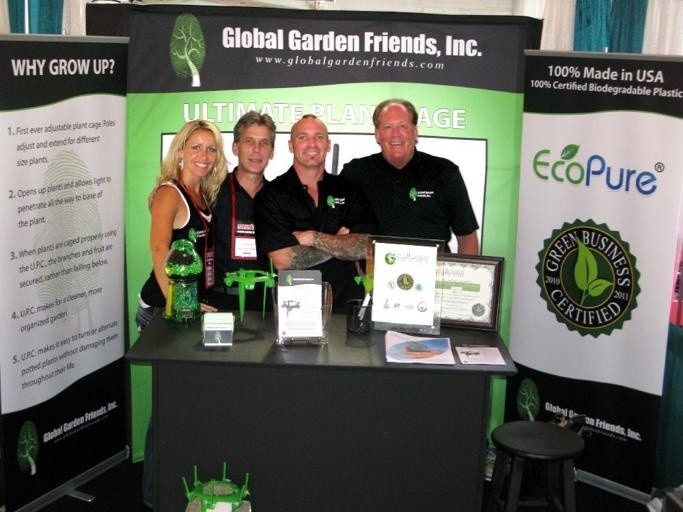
[135,119,232,332]
[253,114,381,315]
[211,112,277,308]
[338,99,480,253]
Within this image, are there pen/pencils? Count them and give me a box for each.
[357,292,371,329]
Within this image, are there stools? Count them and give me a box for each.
[486,419,585,511]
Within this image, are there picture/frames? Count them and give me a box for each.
[364,234,502,335]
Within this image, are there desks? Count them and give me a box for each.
[122,310,519,512]
